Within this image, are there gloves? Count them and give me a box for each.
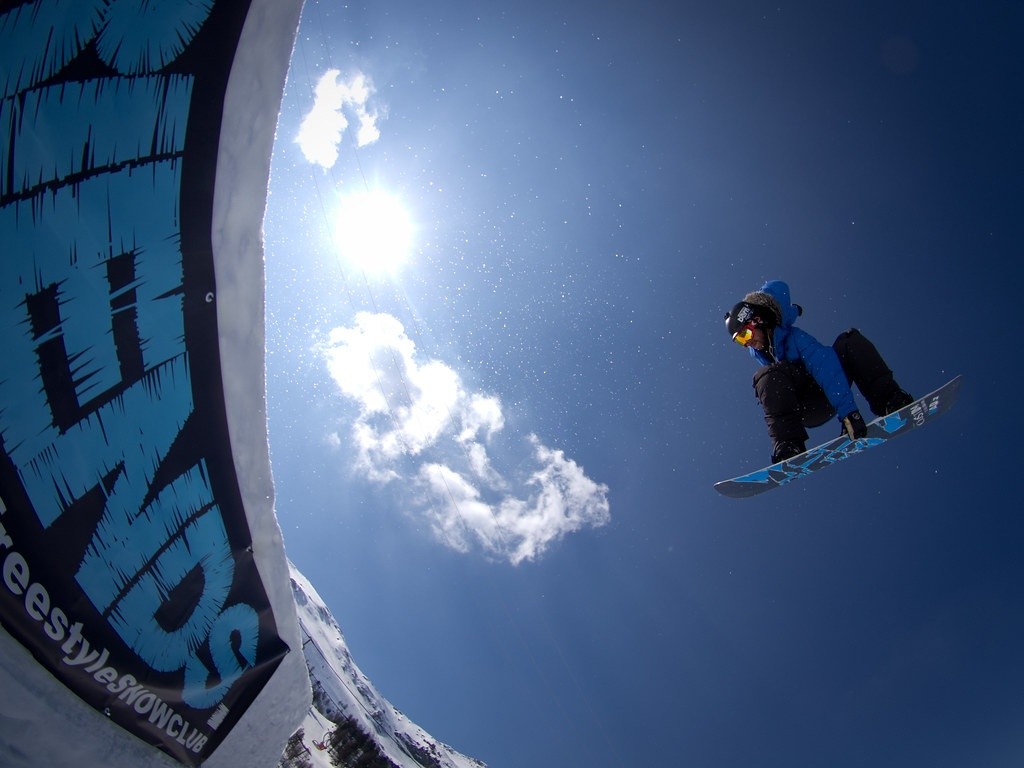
[840,413,867,442]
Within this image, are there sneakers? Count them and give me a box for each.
[771,441,808,464]
[878,391,913,418]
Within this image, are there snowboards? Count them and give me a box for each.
[712,375,966,499]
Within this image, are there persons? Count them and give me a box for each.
[725,279,915,465]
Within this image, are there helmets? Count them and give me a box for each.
[723,301,773,342]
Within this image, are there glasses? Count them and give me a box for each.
[731,321,760,347]
